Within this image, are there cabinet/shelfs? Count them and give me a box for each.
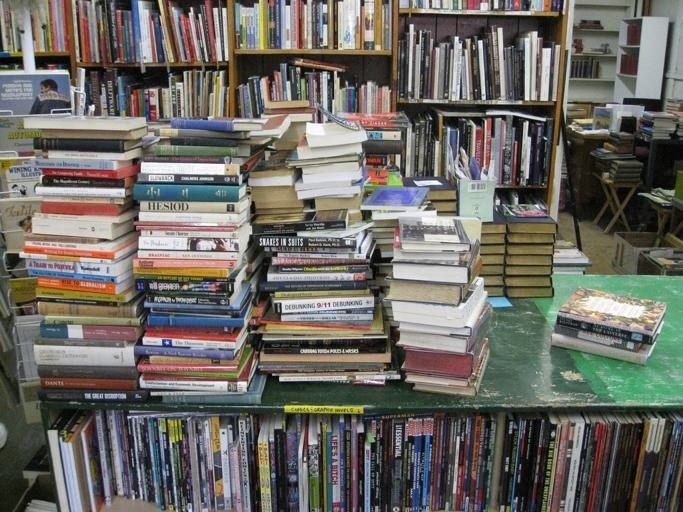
[0,0,396,121]
[394,0,565,222]
[612,15,669,105]
[565,1,631,103]
[36,273,683,512]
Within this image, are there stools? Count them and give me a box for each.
[592,170,643,234]
[662,197,682,248]
[646,197,672,247]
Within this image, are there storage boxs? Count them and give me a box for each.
[610,230,682,275]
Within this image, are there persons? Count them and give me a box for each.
[26,80,72,115]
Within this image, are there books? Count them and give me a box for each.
[73,64,231,116]
[477,203,557,299]
[237,58,392,117]
[405,177,459,216]
[399,20,560,101]
[565,102,597,135]
[405,103,553,187]
[22,114,148,404]
[0,70,73,383]
[552,287,667,363]
[364,187,492,396]
[246,102,399,387]
[1,0,70,58]
[66,0,228,67]
[231,0,394,55]
[552,236,590,275]
[130,118,264,406]
[400,0,562,12]
[588,131,645,182]
[573,58,601,77]
[17,411,682,512]
[639,99,683,141]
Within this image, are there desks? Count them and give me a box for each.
[561,127,608,219]
